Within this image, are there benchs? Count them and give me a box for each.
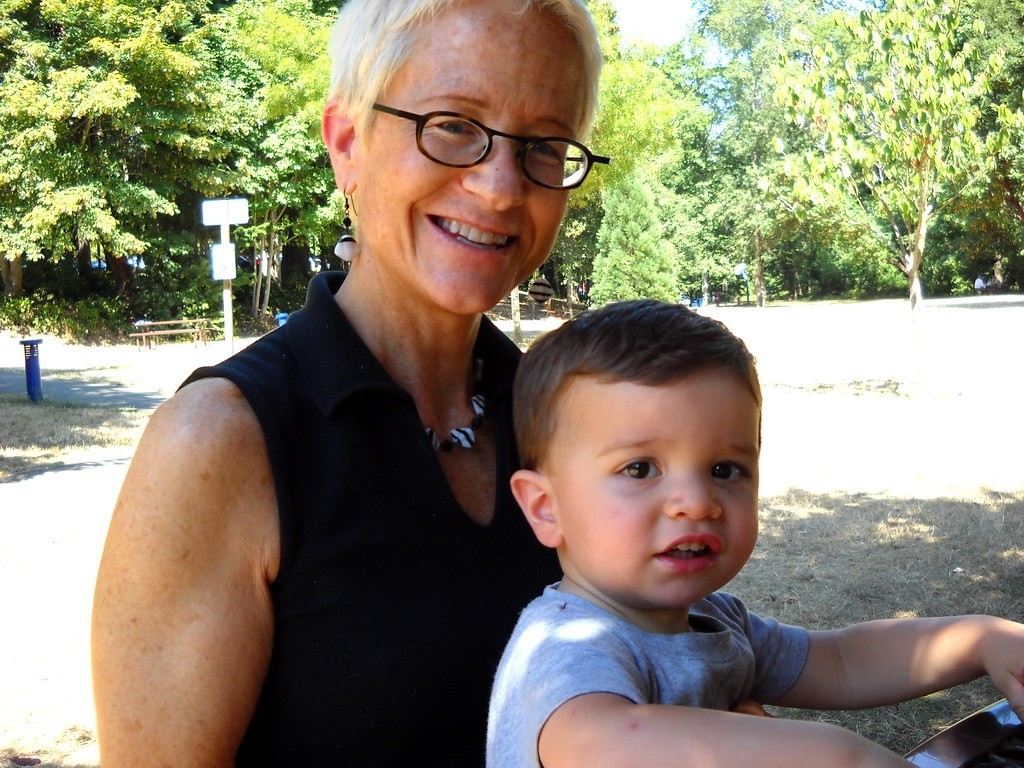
[129,317,211,351]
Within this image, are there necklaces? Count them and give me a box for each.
[421,384,492,449]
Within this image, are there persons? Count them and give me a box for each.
[89,0,606,768]
[486,297,1024,768]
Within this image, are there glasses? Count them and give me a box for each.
[367,103,610,191]
